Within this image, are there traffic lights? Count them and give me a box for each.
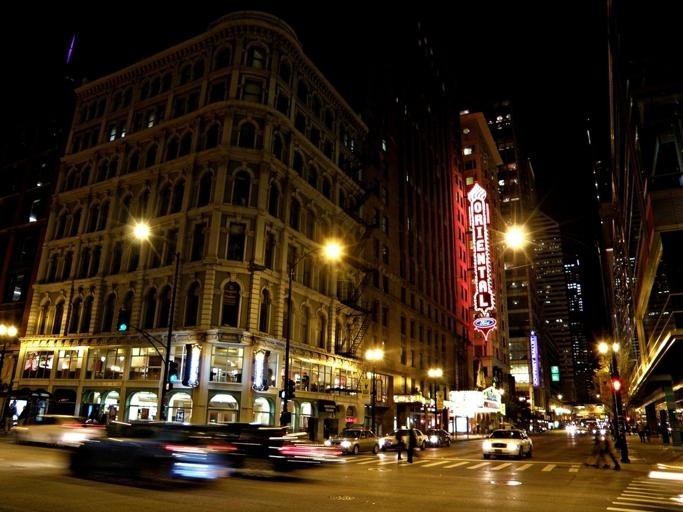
[287,379,295,399]
[116,305,132,333]
[610,376,623,394]
[168,360,178,383]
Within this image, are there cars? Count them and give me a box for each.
[324,429,451,455]
[566,419,611,436]
[482,429,533,459]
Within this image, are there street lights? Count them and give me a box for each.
[280,242,343,425]
[132,221,181,420]
[598,342,630,462]
[366,350,382,435]
[0,324,17,372]
[428,368,442,428]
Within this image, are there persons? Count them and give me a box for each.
[407,423,417,463]
[5,403,16,434]
[302,372,309,391]
[584,431,622,470]
[397,424,404,460]
[638,422,651,443]
